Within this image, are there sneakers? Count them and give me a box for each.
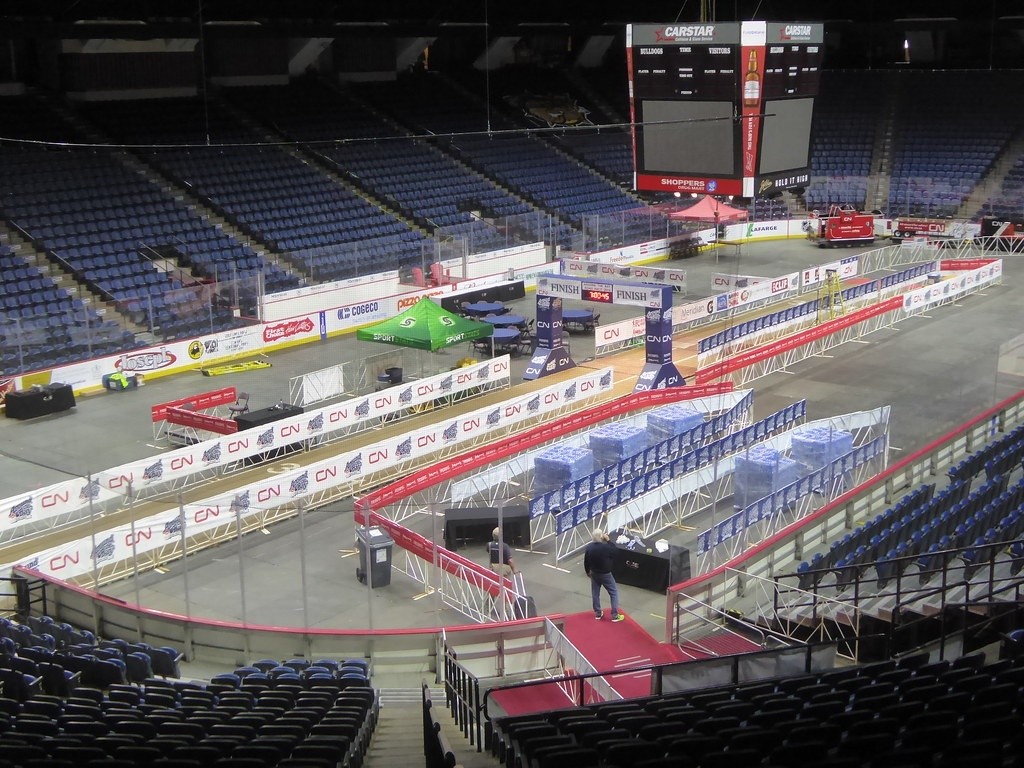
[612,614,624,622]
[595,611,604,620]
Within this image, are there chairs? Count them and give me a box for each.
[0,614,381,768]
[228,392,250,421]
[585,314,600,332]
[794,422,1024,590]
[0,76,448,376]
[448,75,1022,260]
[488,631,1024,768]
[585,305,594,313]
[460,300,534,357]
[375,374,392,393]
[561,331,571,354]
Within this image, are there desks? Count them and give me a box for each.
[466,303,504,327]
[5,382,77,421]
[562,309,593,332]
[442,504,531,552]
[707,239,745,255]
[483,316,525,337]
[479,329,520,354]
[609,532,691,595]
[234,403,304,466]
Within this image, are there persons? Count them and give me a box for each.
[584,529,625,622]
[486,527,522,618]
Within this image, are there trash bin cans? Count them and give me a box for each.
[354,524,396,589]
[385,367,403,384]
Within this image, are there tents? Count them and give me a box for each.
[667,194,750,263]
[355,296,496,394]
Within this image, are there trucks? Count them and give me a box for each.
[891,217,945,238]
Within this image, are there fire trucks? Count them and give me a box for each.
[818,204,875,249]
